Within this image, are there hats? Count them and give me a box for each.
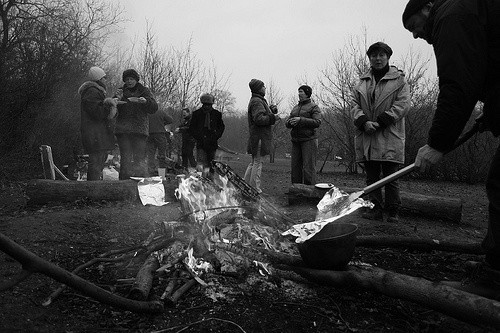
[88,67,107,81]
[249,79,263,92]
[299,85,312,98]
[122,70,140,82]
[403,0,434,27]
[367,42,392,58]
[200,93,214,104]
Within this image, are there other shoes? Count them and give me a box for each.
[363,206,383,220]
[386,209,399,222]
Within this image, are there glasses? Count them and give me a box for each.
[103,76,108,79]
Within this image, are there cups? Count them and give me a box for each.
[158,168,166,177]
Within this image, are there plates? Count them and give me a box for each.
[315,183,334,188]
[118,101,127,104]
[128,98,140,99]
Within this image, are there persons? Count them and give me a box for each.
[78,66,119,181]
[149,110,173,177]
[351,42,411,223]
[401,0,500,303]
[190,93,225,172]
[175,108,196,168]
[113,69,158,180]
[285,85,321,184]
[241,79,280,196]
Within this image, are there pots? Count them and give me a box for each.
[296,222,359,270]
